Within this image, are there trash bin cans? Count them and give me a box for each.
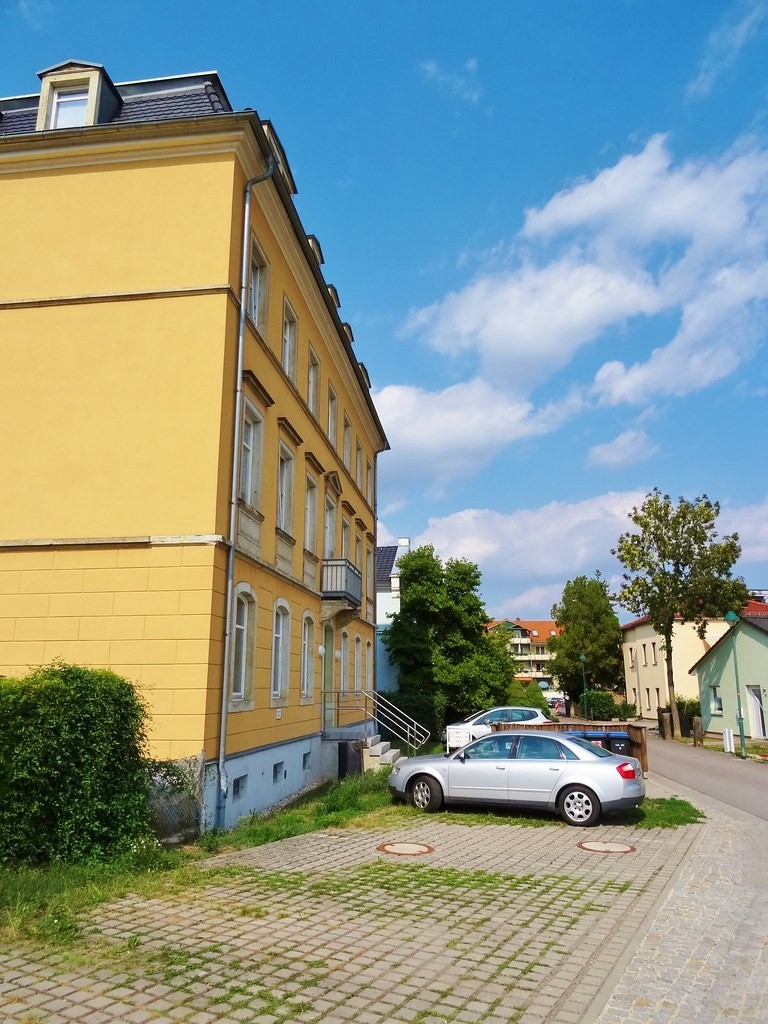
[584,730,608,749]
[566,730,585,738]
[607,733,633,756]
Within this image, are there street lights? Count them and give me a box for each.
[722,611,746,759]
[579,653,588,720]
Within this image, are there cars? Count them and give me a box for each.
[544,695,566,708]
[386,728,646,827]
[440,705,554,749]
